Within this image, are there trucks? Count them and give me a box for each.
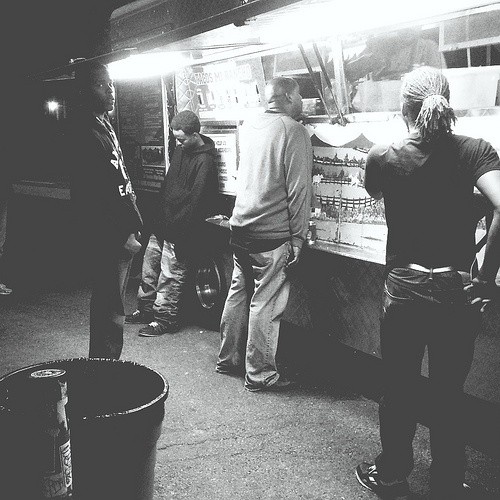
[1,0,500,404]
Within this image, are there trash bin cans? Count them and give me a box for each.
[0,357,168,500]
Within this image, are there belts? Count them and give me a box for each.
[409,263,454,274]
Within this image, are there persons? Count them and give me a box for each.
[214,77,316,394]
[70,61,144,360]
[355,63,500,492]
[123,110,219,338]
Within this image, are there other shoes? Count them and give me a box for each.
[124,309,155,324]
[215,364,245,374]
[355,462,410,500]
[139,320,179,337]
[244,376,296,393]
